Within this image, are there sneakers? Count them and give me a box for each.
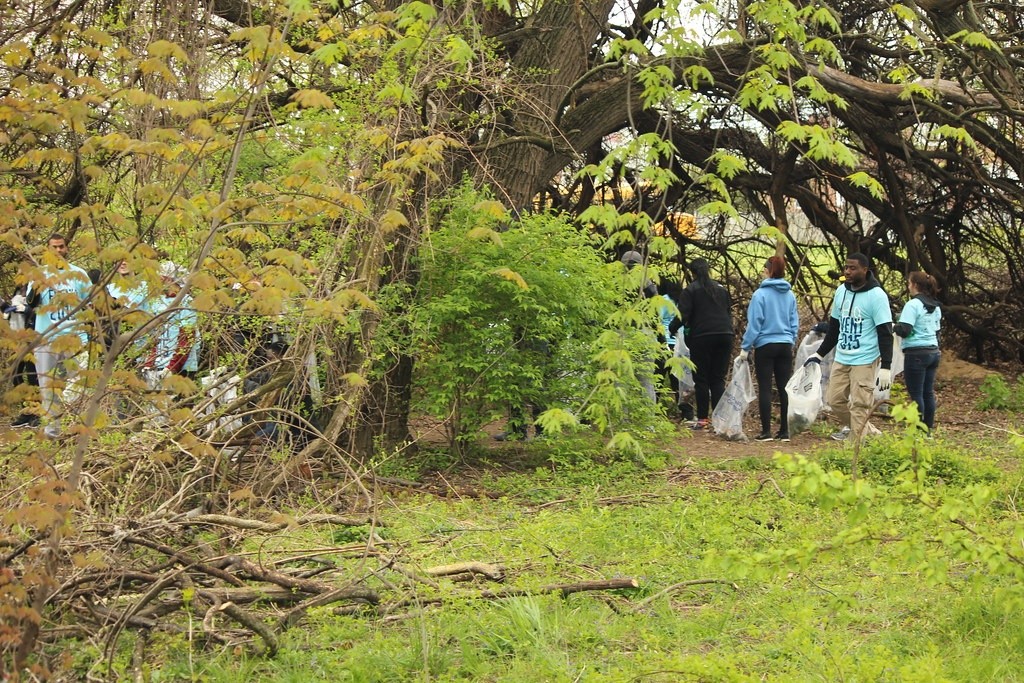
[754,432,774,442]
[774,430,791,442]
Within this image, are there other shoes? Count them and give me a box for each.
[693,418,710,433]
[493,429,550,445]
[831,432,849,440]
[842,426,853,434]
[10,418,31,428]
[27,420,44,429]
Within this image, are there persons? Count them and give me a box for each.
[804,254,894,443]
[739,256,800,442]
[892,272,942,429]
[809,322,851,441]
[0,235,735,472]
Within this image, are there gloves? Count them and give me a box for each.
[803,353,824,365]
[875,367,904,393]
[735,349,749,367]
[669,329,679,340]
